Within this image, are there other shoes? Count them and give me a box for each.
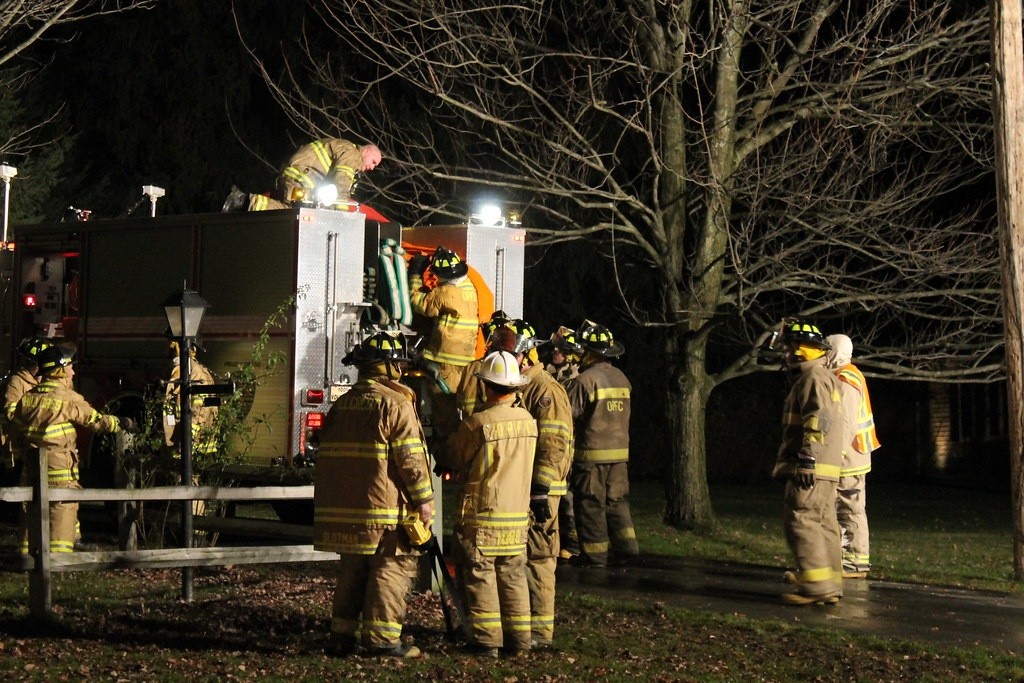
[841,564,870,579]
[559,550,640,569]
[780,589,838,605]
[74,541,98,552]
[454,623,551,659]
[369,642,423,661]
[329,630,362,649]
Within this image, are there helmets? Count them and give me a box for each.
[779,318,831,351]
[341,331,412,366]
[16,336,53,364]
[32,341,77,378]
[432,245,469,279]
[163,321,206,352]
[472,310,626,388]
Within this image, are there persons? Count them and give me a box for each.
[823,334,882,580]
[218,136,383,212]
[568,319,641,568]
[770,320,845,606]
[0,336,57,517]
[544,324,581,562]
[435,351,532,658]
[409,250,480,438]
[485,319,574,652]
[12,339,136,572]
[313,331,435,659]
[162,323,217,539]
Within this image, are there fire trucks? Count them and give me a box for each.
[0,193,526,546]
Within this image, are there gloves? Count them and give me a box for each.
[530,484,552,524]
[118,416,134,432]
[793,454,817,492]
[408,254,430,274]
